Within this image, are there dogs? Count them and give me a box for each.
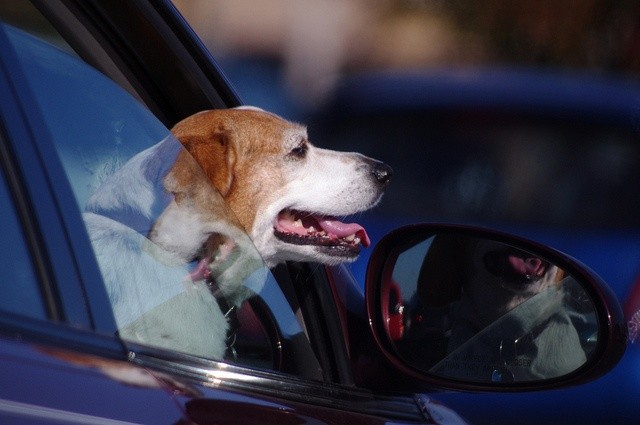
[83,105,395,365]
